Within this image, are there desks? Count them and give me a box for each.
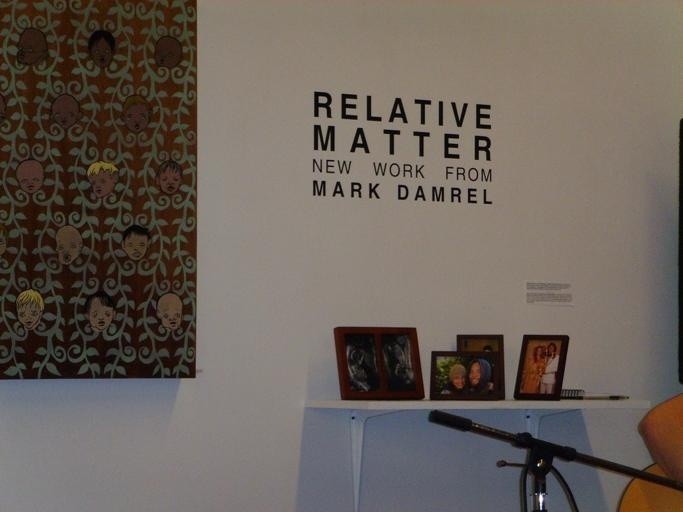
[305,395,651,512]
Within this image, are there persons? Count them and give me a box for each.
[539,343,560,394]
[464,359,494,395]
[440,363,494,395]
[522,345,546,393]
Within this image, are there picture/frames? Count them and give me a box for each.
[333,325,569,404]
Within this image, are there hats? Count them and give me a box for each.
[449,363,467,381]
